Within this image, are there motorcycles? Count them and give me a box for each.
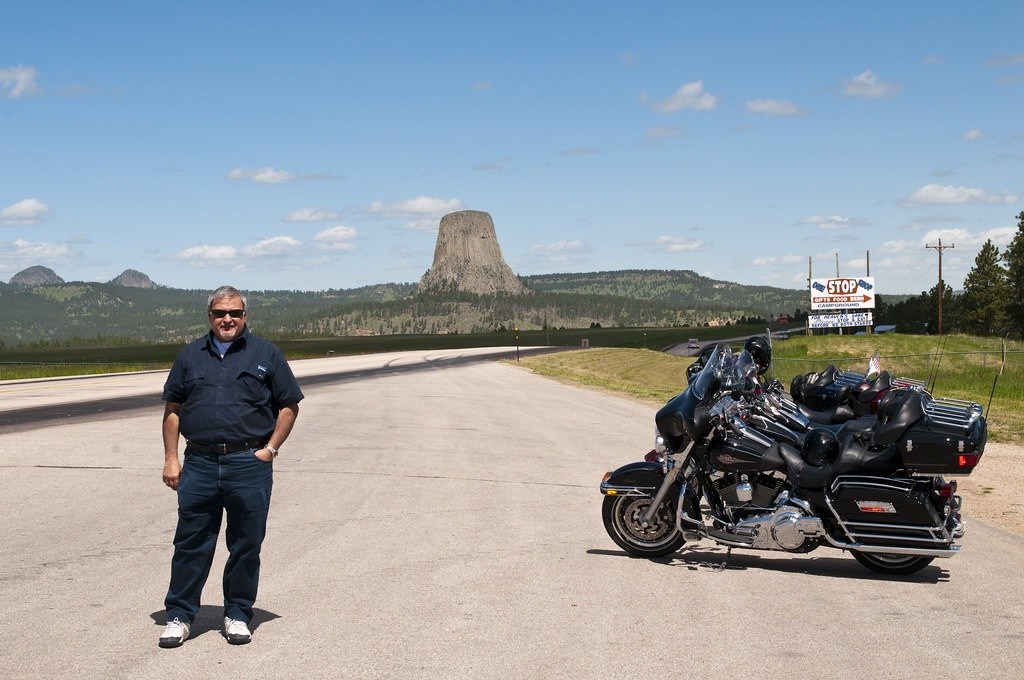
[600,336,987,576]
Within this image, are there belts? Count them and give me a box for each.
[187,437,268,456]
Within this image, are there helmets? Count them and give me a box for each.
[801,427,841,468]
[744,337,771,374]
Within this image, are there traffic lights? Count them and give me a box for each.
[514,327,520,340]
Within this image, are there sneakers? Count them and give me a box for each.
[159,617,190,648]
[222,616,251,645]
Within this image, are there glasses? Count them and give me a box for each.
[210,308,246,318]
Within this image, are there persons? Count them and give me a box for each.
[159,286,305,648]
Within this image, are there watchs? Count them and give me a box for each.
[265,445,278,457]
